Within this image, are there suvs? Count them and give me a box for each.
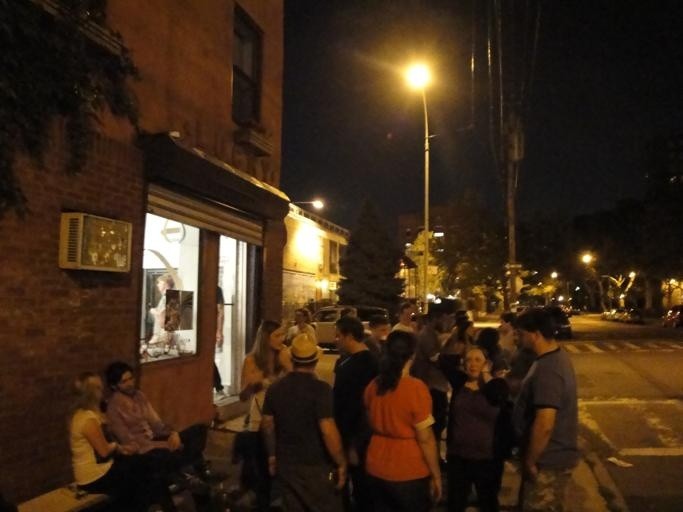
[542,305,572,338]
[659,305,683,328]
[286,304,392,351]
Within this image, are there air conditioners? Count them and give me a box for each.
[59,212,132,275]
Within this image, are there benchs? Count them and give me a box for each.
[15,483,109,512]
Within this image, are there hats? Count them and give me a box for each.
[288,333,321,363]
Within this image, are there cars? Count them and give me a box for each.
[600,306,641,323]
[516,300,579,317]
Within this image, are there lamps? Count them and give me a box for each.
[289,196,325,211]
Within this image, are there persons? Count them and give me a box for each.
[393,298,537,471]
[440,309,509,511]
[67,361,207,512]
[149,272,175,348]
[213,285,224,395]
[509,309,578,511]
[332,316,383,511]
[239,319,287,510]
[288,308,314,345]
[362,329,441,511]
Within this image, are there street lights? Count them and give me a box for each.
[293,200,323,210]
[425,213,447,244]
[399,51,433,318]
